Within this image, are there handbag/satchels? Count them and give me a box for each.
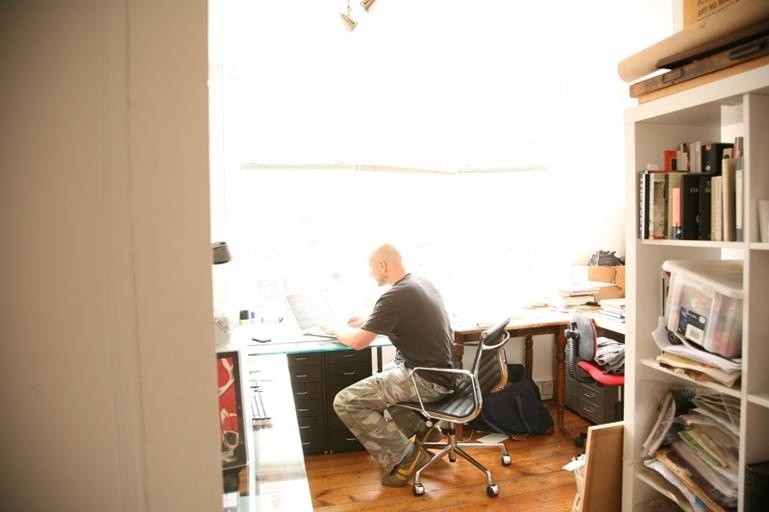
[463,378,555,437]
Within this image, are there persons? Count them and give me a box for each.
[317,241,457,488]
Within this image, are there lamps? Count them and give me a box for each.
[212,241,232,264]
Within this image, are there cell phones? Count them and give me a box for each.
[252,336,271,343]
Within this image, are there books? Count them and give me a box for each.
[537,282,627,324]
[641,384,741,512]
[654,268,745,389]
[637,133,768,244]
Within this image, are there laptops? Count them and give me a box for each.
[285,288,344,338]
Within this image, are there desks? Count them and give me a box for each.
[452,307,569,443]
[215,329,393,512]
[558,287,627,346]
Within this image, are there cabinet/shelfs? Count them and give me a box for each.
[622,72,769,507]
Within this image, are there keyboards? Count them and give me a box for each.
[251,390,273,427]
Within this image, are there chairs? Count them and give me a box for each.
[564,317,628,445]
[388,318,511,497]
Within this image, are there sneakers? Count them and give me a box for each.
[381,445,431,487]
[414,422,445,445]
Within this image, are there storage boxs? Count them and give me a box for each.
[662,255,745,360]
[682,0,741,31]
